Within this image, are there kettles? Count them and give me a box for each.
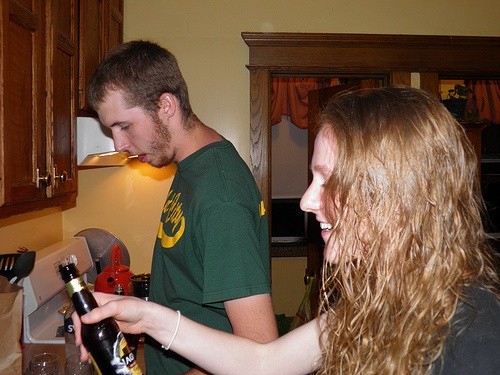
[94,244,135,296]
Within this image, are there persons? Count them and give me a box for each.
[87,40,280,375]
[69,85,500,375]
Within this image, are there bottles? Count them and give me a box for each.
[58,262,144,375]
[65,303,94,375]
[112,283,140,359]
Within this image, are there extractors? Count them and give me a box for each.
[76,116,134,168]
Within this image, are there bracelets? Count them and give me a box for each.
[161,309,181,350]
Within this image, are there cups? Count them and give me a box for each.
[28,352,62,375]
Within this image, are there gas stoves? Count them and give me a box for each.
[17,236,95,345]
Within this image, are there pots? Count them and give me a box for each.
[128,272,151,301]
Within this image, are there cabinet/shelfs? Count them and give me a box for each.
[0,0,124,227]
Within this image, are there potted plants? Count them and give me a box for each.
[441,83,485,122]
